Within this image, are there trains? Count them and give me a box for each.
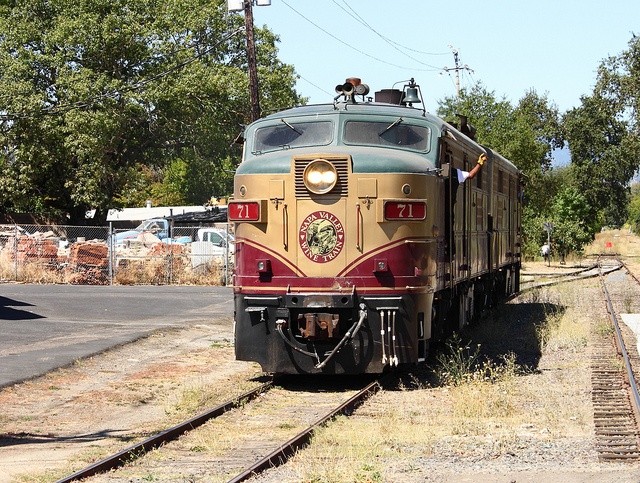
[227,75,525,381]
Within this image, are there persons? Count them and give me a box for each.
[542,242,550,261]
[445,152,487,261]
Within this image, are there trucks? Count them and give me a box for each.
[192,226,235,252]
[109,218,192,244]
